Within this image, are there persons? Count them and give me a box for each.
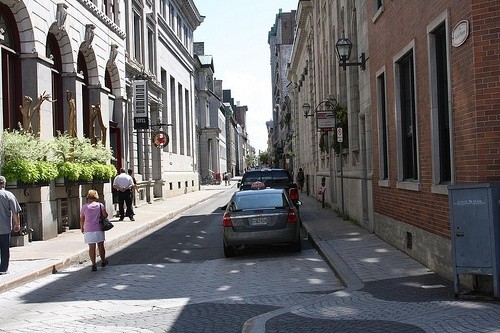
[296,167,305,193]
[126,169,141,217]
[217,165,273,186]
[0,176,22,275]
[113,168,135,221]
[116,172,127,217]
[80,189,109,271]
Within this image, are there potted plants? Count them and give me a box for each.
[0,128,118,189]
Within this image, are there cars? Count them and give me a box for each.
[220,182,303,258]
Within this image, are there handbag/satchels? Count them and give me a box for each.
[99,202,114,232]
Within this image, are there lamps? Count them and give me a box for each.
[302,103,314,118]
[334,38,365,71]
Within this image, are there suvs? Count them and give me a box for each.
[237,167,301,209]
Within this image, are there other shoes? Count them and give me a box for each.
[92,265,97,271]
[101,260,108,267]
